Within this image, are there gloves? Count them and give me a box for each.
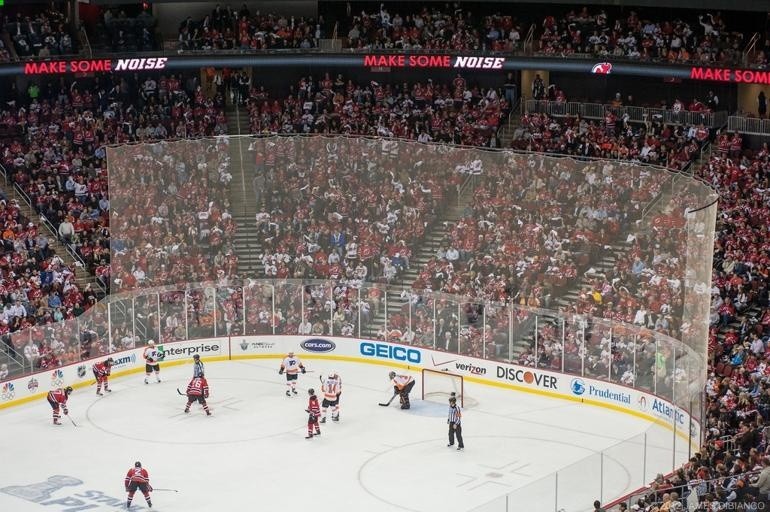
[301,367,305,373]
[64,409,68,415]
[279,368,284,374]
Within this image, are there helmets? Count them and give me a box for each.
[329,370,340,378]
[108,357,113,366]
[193,355,200,359]
[135,462,141,467]
[389,371,396,378]
[198,372,204,377]
[309,388,314,395]
[148,340,155,348]
[66,387,73,395]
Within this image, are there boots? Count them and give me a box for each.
[105,385,112,391]
[147,500,152,507]
[285,390,291,396]
[54,418,62,425]
[127,501,131,507]
[292,388,297,393]
[96,388,103,395]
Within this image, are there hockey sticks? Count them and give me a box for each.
[152,489,177,492]
[67,412,83,426]
[379,395,396,406]
[177,388,188,395]
